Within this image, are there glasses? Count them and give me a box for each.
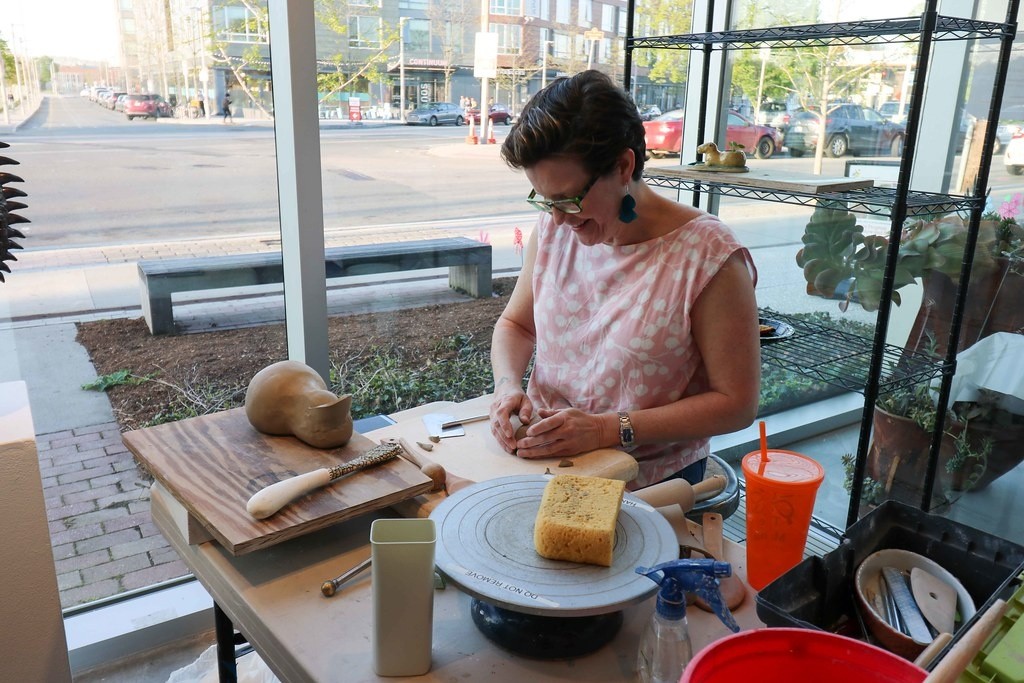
[526,169,604,214]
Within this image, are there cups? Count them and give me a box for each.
[855,550,977,655]
[370,519,437,677]
[741,450,823,591]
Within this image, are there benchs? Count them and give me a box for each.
[136,236,492,335]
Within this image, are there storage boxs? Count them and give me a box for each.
[755,500,1024,683]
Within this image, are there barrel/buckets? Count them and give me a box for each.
[678,627,933,683]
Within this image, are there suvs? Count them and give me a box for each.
[878,100,910,128]
[125,93,174,120]
[757,101,803,131]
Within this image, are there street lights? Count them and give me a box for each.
[189,6,211,120]
[400,16,414,123]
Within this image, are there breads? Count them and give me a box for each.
[534,473,627,567]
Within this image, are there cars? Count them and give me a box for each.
[955,105,1024,176]
[784,101,906,159]
[80,85,129,112]
[466,103,514,125]
[405,102,466,127]
[642,108,784,160]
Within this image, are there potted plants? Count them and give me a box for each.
[686,141,749,172]
[797,175,1024,516]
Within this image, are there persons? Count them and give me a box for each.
[637,103,661,121]
[222,93,233,123]
[8,90,13,109]
[198,90,205,117]
[489,97,494,104]
[459,96,476,109]
[489,69,761,492]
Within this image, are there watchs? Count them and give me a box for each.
[617,411,634,448]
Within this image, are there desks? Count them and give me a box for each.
[150,392,766,683]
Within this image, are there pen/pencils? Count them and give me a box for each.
[441,414,490,429]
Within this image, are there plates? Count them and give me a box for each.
[758,316,795,345]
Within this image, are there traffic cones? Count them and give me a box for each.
[466,114,478,145]
[485,118,496,144]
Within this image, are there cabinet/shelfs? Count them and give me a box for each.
[623,0,1020,562]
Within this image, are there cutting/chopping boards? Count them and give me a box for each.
[121,406,433,558]
[350,400,639,520]
[644,162,874,194]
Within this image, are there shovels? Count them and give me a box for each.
[694,512,747,613]
